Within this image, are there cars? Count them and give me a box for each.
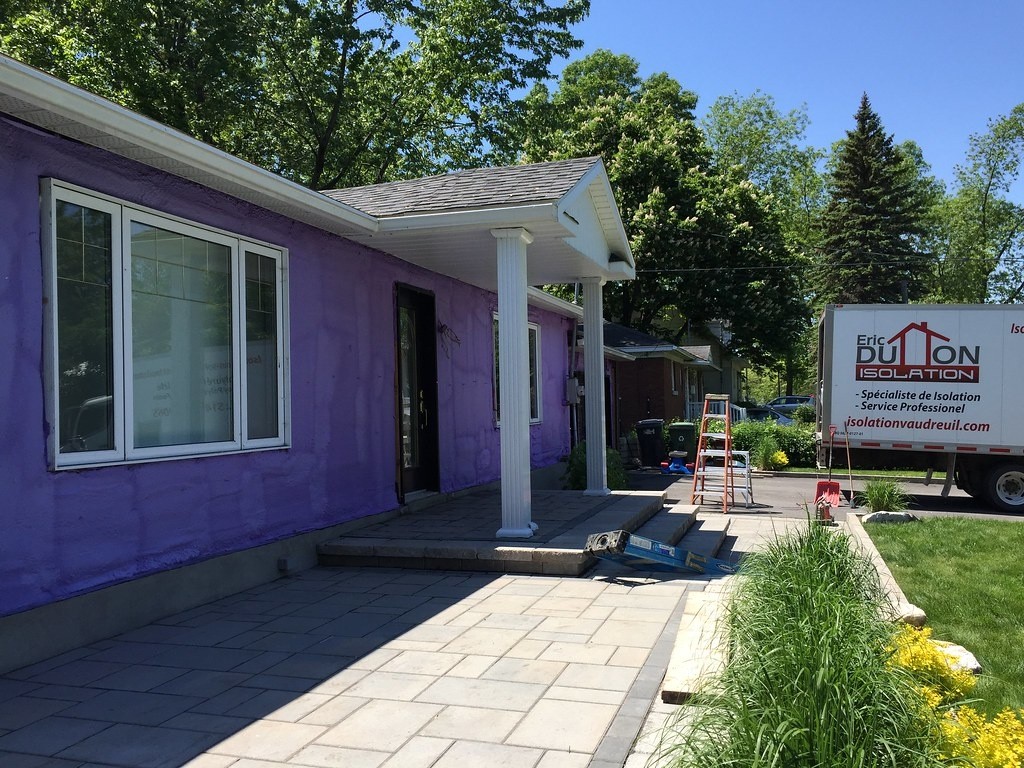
[744,406,797,426]
[767,396,814,413]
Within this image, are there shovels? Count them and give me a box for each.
[813,424,840,508]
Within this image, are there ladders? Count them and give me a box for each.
[691,393,736,513]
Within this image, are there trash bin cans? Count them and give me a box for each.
[668,421,696,466]
[634,418,665,467]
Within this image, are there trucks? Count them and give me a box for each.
[815,302,1024,515]
